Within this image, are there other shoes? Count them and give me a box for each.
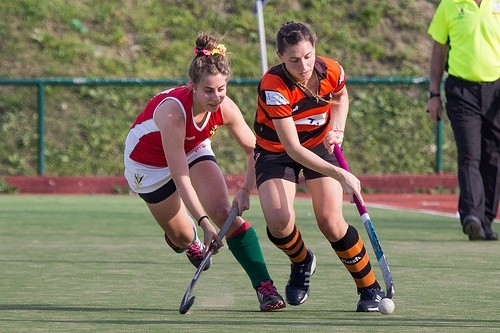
[463,215,486,240]
[484,225,498,239]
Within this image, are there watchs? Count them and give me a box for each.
[428,91,440,99]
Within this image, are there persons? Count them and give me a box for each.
[253,20,385,312]
[427,0,500,241]
[124,31,286,311]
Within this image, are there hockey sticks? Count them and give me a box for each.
[178,203,240,315]
[331,142,396,300]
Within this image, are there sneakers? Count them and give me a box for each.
[257,279,286,311]
[185,213,211,271]
[356,288,385,312]
[286,247,316,305]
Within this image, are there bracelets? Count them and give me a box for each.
[239,186,251,194]
[198,215,209,226]
[332,129,344,132]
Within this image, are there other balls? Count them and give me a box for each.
[378,297,395,315]
